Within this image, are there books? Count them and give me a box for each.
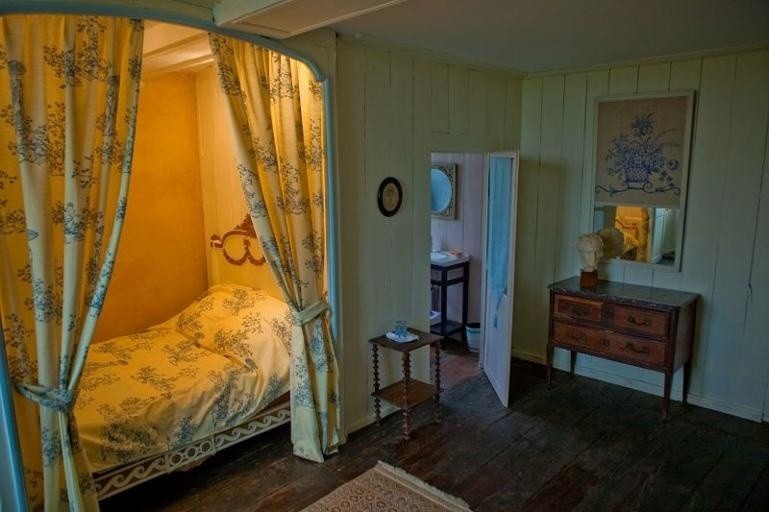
[430,311,441,326]
[431,251,456,263]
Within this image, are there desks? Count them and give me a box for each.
[546,275,701,422]
[430,261,469,349]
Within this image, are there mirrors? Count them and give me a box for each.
[582,87,696,273]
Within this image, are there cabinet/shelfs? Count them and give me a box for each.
[367,327,444,441]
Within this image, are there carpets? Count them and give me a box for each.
[298,460,475,512]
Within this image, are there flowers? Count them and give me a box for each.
[599,228,624,256]
[577,235,601,272]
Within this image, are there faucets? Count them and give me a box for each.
[430,236,433,252]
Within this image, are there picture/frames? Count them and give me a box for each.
[377,177,402,217]
[430,164,457,220]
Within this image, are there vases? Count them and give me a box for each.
[579,269,597,290]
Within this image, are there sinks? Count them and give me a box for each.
[430,250,470,267]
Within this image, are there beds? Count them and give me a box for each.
[0,0,346,512]
